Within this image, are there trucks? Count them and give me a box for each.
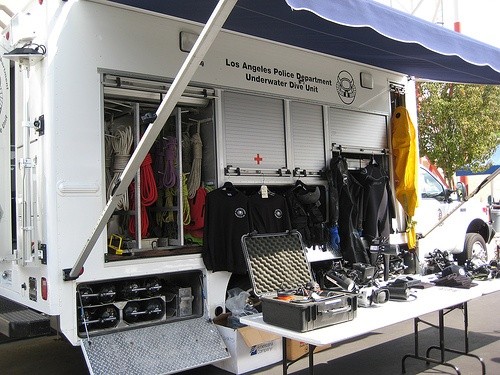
[0,0,422,375]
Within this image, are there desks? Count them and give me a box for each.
[239,269,500,375]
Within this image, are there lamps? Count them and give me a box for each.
[3,48,42,61]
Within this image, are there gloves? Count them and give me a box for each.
[330,226,341,252]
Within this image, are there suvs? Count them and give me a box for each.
[418,162,496,269]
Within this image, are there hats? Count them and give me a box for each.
[442,263,466,279]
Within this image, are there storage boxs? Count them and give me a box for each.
[286,338,332,361]
[209,312,285,375]
[241,230,358,332]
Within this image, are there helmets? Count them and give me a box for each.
[296,185,320,205]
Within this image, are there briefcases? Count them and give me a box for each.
[240,229,358,333]
